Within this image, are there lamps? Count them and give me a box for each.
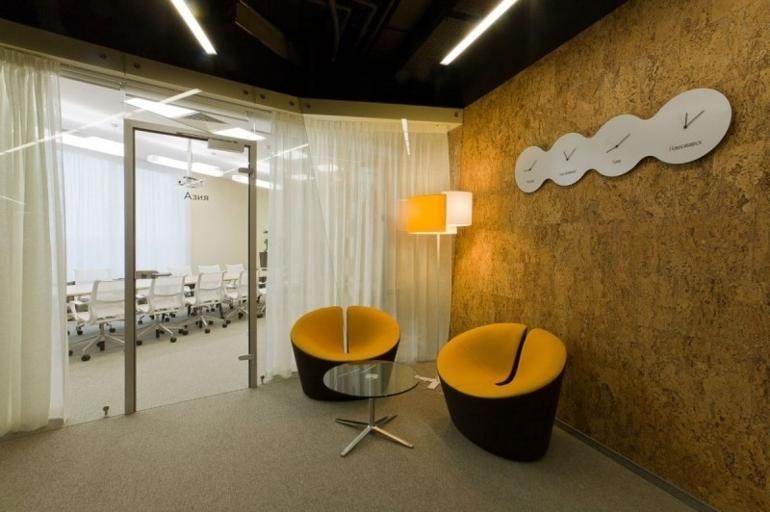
[399,190,473,391]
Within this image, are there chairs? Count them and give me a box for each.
[287,302,402,400]
[435,321,569,462]
[64,262,271,359]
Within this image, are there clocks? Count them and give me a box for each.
[513,87,732,195]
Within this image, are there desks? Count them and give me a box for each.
[323,359,423,457]
